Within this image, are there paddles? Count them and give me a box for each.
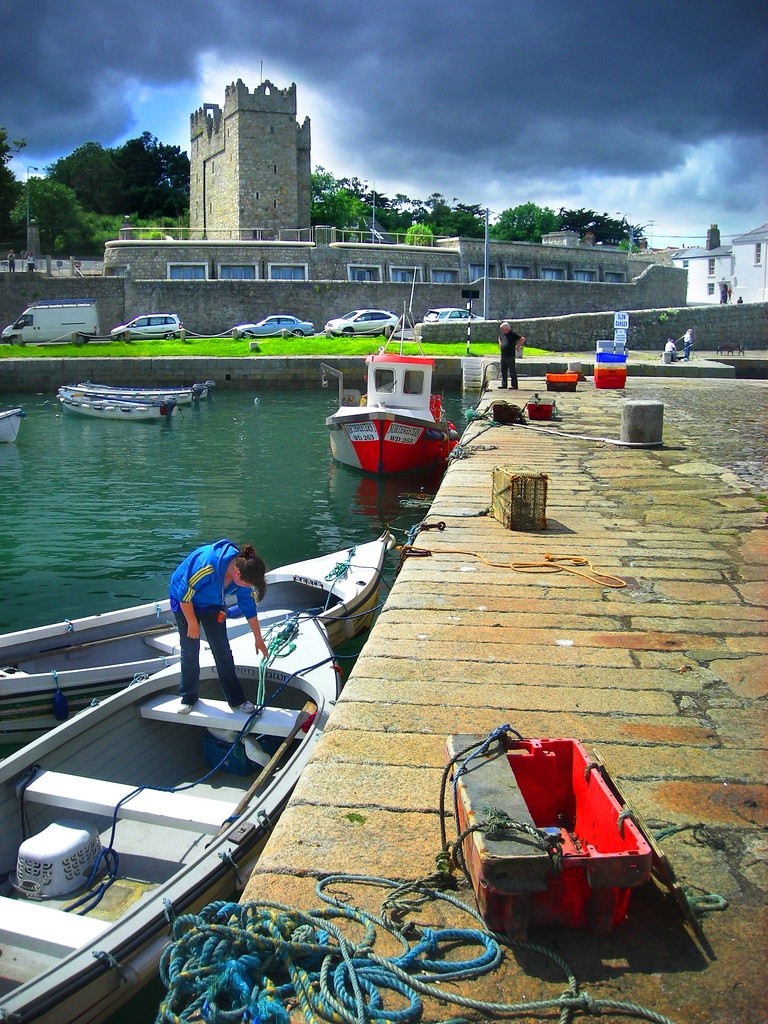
[203,698,316,850]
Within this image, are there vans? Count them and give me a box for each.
[2,297,103,343]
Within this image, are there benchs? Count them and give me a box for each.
[0,895,112,952]
[717,344,745,357]
[16,769,238,835]
[146,629,211,655]
[139,693,307,740]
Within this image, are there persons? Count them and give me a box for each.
[169,539,269,716]
[720,286,744,305]
[7,250,16,272]
[26,251,36,271]
[498,322,525,390]
[665,329,694,361]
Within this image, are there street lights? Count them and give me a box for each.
[26,165,39,225]
[615,210,633,254]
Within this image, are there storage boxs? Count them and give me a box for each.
[493,403,519,420]
[9,818,112,898]
[444,738,653,934]
[528,398,557,420]
[198,729,282,777]
[546,374,578,391]
[594,341,629,388]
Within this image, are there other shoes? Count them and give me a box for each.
[178,704,193,714]
[238,701,255,713]
[498,386,507,389]
[509,387,518,390]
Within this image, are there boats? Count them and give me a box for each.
[0,407,23,443]
[323,353,458,475]
[57,388,180,420]
[60,383,211,406]
[2,615,347,1024]
[1,527,386,754]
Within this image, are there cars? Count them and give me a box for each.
[232,315,316,340]
[323,308,403,338]
[422,307,483,324]
[109,313,180,340]
